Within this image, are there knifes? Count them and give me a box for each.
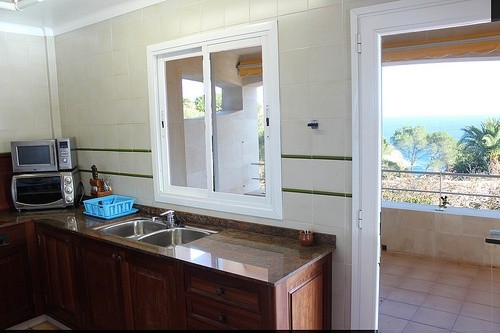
[91,165,98,180]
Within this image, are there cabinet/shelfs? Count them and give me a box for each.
[0,219,332,333]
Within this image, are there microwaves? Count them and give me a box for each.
[10,137,77,173]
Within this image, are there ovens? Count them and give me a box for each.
[11,169,80,213]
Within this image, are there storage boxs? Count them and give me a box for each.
[83,195,135,217]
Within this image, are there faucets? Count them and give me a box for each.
[152,210,174,228]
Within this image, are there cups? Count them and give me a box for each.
[299,230,313,246]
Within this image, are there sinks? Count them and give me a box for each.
[93,216,164,242]
[137,225,218,251]
